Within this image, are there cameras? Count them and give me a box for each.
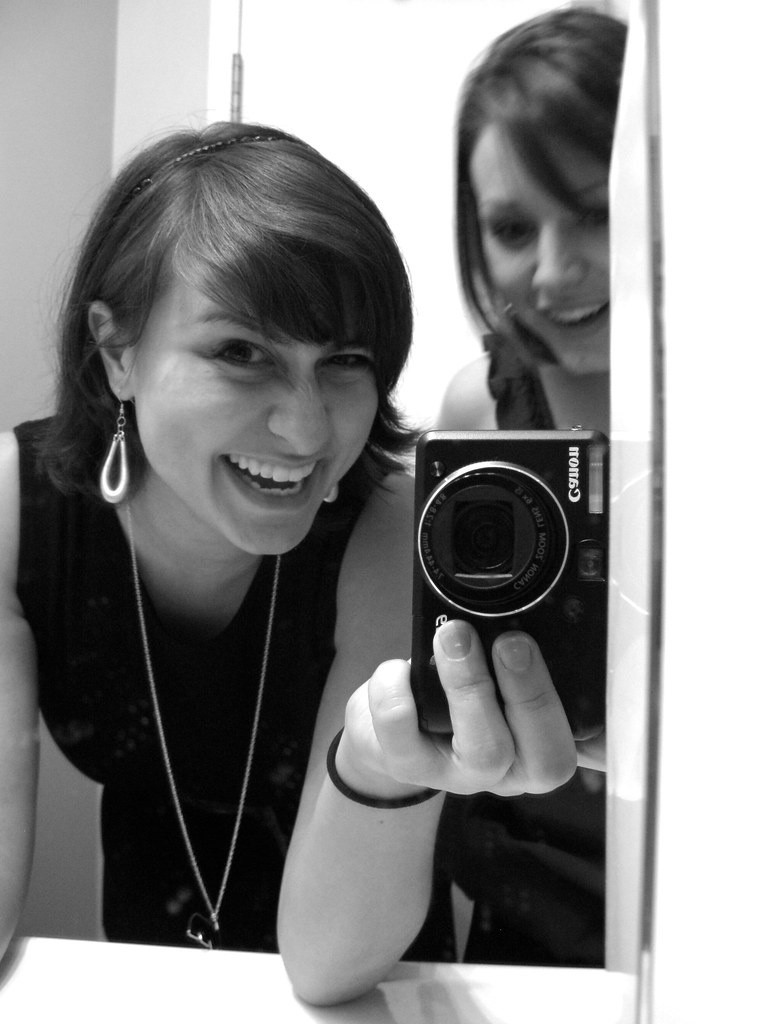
[409,426,612,740]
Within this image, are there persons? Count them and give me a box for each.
[0,122,578,1007]
[436,10,628,969]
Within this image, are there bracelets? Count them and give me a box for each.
[326,726,442,809]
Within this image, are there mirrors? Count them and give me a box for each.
[0,0,768,1024]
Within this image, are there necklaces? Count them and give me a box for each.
[126,496,281,950]
